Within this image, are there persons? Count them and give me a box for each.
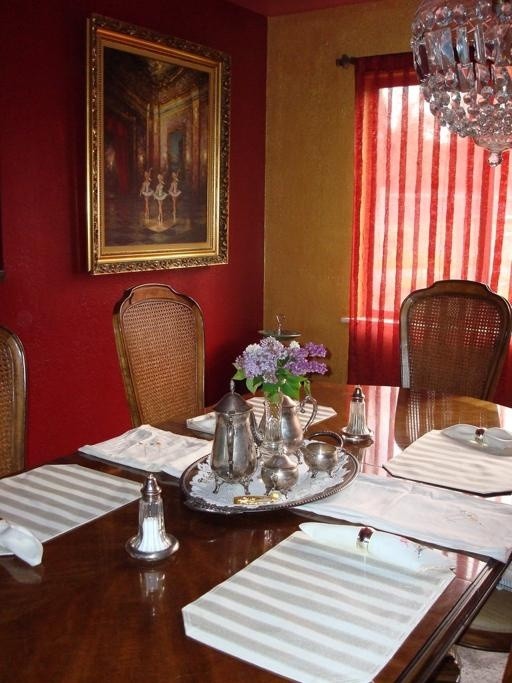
[139,168,181,230]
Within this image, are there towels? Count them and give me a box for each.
[441,424,512,449]
[299,522,457,572]
[186,411,218,435]
[0,517,44,567]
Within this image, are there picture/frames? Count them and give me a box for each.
[83,13,232,276]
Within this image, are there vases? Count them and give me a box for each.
[261,391,287,454]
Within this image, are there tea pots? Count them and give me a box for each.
[212,380,257,496]
[259,388,317,464]
[300,430,343,478]
[261,452,303,499]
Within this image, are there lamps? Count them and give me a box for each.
[410,1,512,167]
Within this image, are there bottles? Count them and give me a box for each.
[339,439,373,474]
[128,472,179,560]
[342,385,377,443]
[127,551,176,622]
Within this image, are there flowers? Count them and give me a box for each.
[231,336,327,405]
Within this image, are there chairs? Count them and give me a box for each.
[0,324,29,480]
[399,280,512,400]
[456,587,512,683]
[113,284,207,429]
